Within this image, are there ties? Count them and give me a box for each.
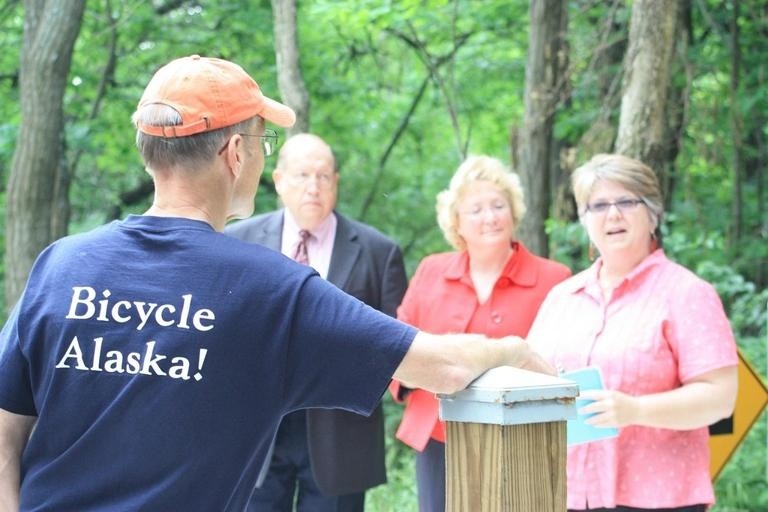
[293,229,312,269]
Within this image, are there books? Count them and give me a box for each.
[558,367,619,445]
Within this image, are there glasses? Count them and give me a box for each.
[583,198,645,214]
[215,128,278,159]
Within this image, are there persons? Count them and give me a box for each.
[387,150,572,512]
[524,152,738,512]
[0,53,553,512]
[226,132,409,512]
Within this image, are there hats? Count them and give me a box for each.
[131,53,297,139]
